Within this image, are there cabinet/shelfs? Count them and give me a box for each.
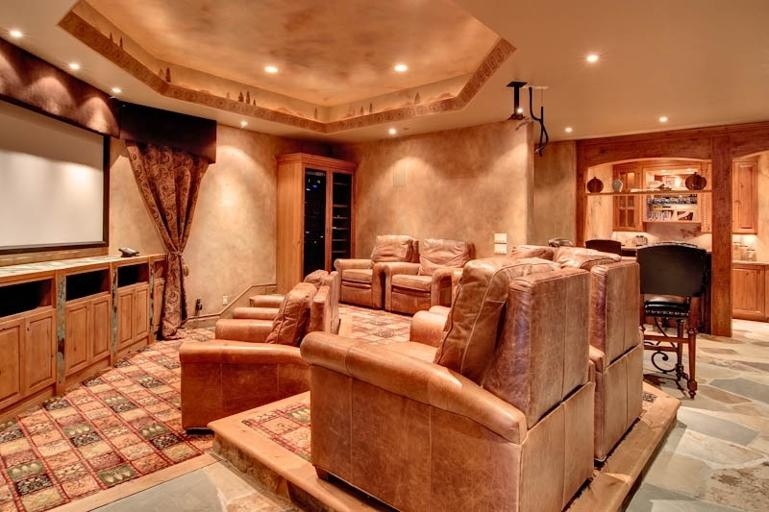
[611,161,643,232]
[1,281,150,427]
[700,158,759,235]
[276,152,358,296]
[730,262,768,321]
[644,161,703,223]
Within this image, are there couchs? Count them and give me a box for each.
[300,245,642,510]
[334,235,474,314]
[180,270,343,433]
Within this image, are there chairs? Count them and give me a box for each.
[633,240,712,400]
